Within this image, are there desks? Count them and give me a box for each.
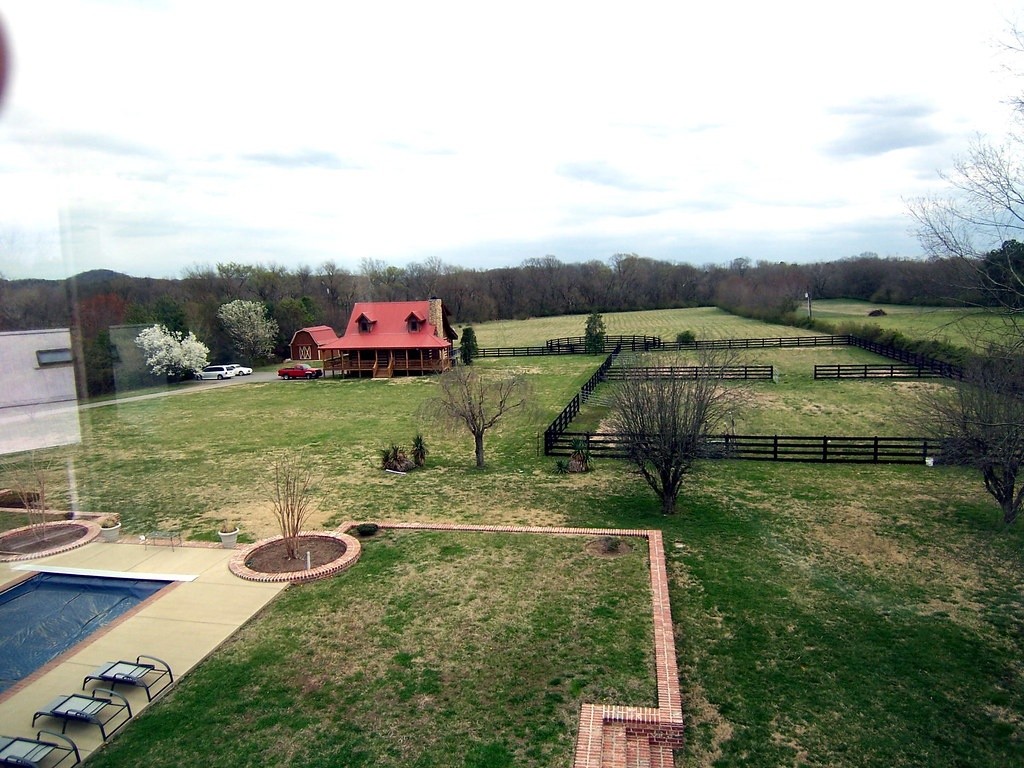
[145,531,182,552]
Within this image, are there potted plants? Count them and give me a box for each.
[218,515,239,548]
[99,513,121,540]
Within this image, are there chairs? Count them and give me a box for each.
[83,654,174,702]
[32,688,132,742]
[0,730,81,768]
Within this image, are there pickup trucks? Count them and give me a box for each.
[277,363,323,380]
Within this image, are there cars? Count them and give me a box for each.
[231,364,253,376]
[193,365,235,380]
